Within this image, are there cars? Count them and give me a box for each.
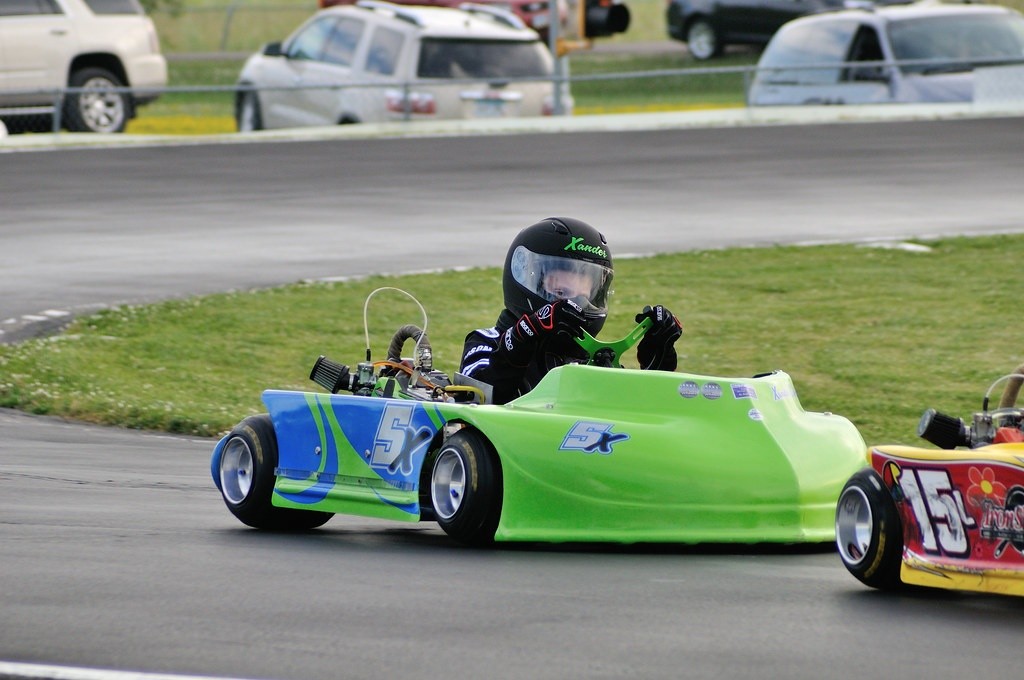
[748,2,1024,107]
[324,0,630,43]
[665,1,946,60]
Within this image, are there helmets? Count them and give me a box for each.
[502,216,614,360]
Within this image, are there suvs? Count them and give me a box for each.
[0,0,169,135]
[233,2,570,133]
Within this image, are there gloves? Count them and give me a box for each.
[512,298,587,355]
[635,305,683,370]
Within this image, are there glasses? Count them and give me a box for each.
[554,286,590,309]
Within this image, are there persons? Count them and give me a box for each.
[461,217,682,404]
